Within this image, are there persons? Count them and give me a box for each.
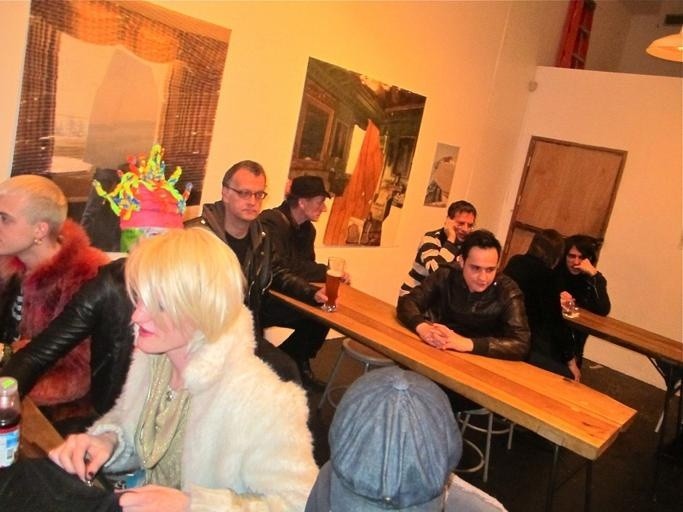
[1,173,112,408]
[303,365,509,512]
[429,156,454,202]
[396,200,478,319]
[0,144,194,432]
[396,228,612,412]
[80,162,140,252]
[47,227,321,512]
[181,158,342,386]
[259,174,354,395]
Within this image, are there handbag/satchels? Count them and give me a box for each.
[0,456,123,512]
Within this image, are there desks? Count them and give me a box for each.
[17,394,66,461]
[561,303,683,461]
[264,280,639,512]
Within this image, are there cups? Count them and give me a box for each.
[321,256,346,313]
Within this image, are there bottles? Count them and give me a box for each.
[0,377,22,469]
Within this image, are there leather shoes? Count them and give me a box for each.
[302,361,327,390]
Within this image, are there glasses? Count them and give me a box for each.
[227,186,267,200]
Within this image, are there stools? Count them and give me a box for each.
[317,336,397,414]
[453,407,517,482]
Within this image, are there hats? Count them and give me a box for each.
[305,366,463,512]
[285,176,330,200]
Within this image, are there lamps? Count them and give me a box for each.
[646,24,683,62]
